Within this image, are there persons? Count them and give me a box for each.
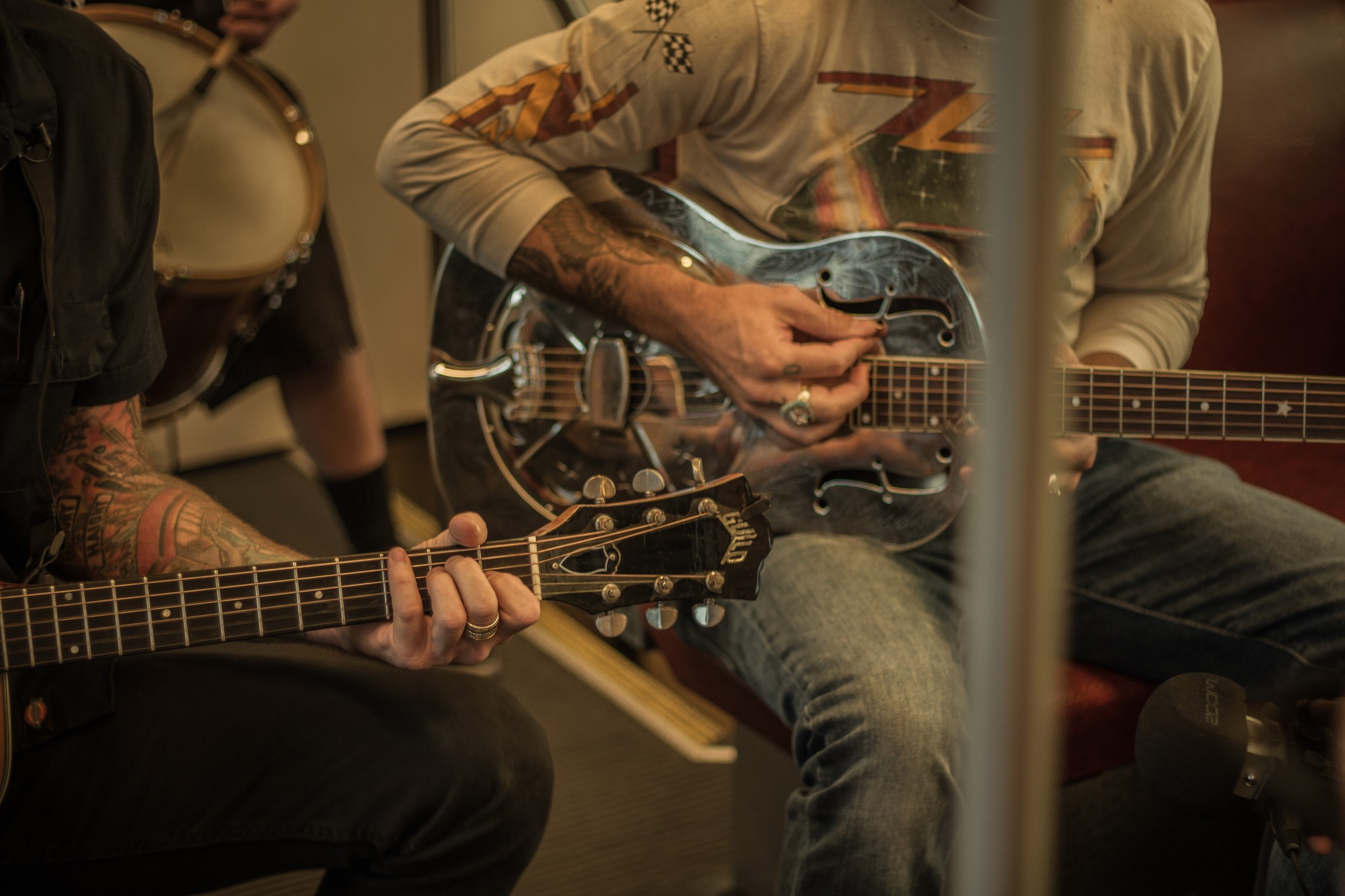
[4,0,553,896]
[372,0,1345,896]
[113,0,398,558]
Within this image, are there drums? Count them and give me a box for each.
[73,6,326,402]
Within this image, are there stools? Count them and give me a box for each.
[625,594,1155,894]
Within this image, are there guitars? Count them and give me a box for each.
[0,452,771,665]
[424,164,1345,545]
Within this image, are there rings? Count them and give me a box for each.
[778,383,816,427]
[463,613,500,640]
